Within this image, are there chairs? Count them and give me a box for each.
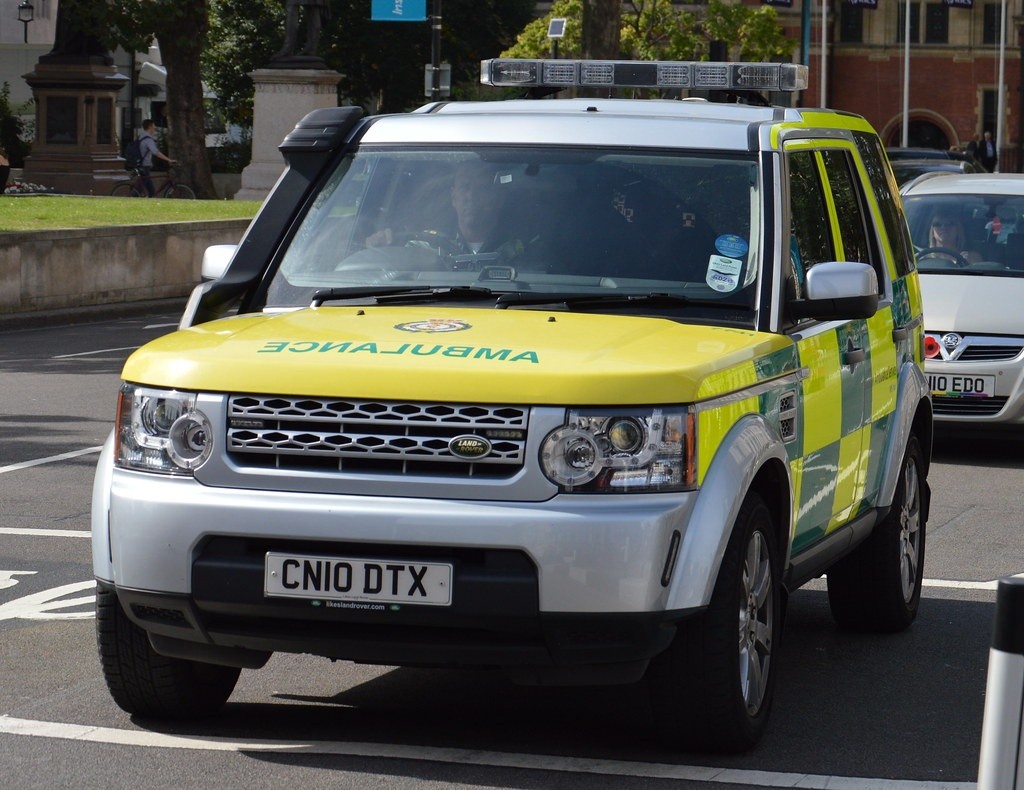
[920,203,987,262]
[659,178,756,279]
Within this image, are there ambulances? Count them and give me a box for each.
[91,59,934,755]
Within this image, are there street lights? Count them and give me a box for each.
[18,0,34,43]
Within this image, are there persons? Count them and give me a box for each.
[365,162,547,270]
[967,131,997,173]
[132,119,178,196]
[920,210,981,264]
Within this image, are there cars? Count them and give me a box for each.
[885,147,988,188]
[896,172,1024,425]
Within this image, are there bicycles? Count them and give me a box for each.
[110,163,196,199]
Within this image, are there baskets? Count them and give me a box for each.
[173,164,191,185]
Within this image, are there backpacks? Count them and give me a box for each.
[124,135,150,168]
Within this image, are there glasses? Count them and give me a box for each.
[933,221,956,228]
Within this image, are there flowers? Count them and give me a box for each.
[4,179,48,194]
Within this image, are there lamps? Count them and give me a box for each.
[17,0,33,43]
